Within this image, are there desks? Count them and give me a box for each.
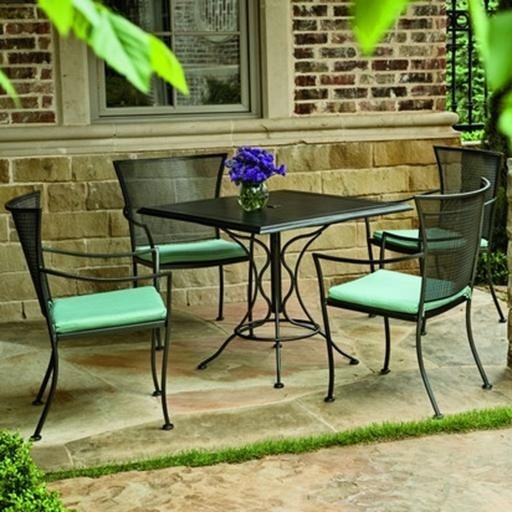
[136,190,415,388]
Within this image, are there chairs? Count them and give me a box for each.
[311,176,492,420]
[3,189,173,442]
[113,151,250,350]
[364,142,507,335]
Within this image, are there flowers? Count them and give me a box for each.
[224,143,287,185]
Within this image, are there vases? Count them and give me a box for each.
[236,181,269,213]
